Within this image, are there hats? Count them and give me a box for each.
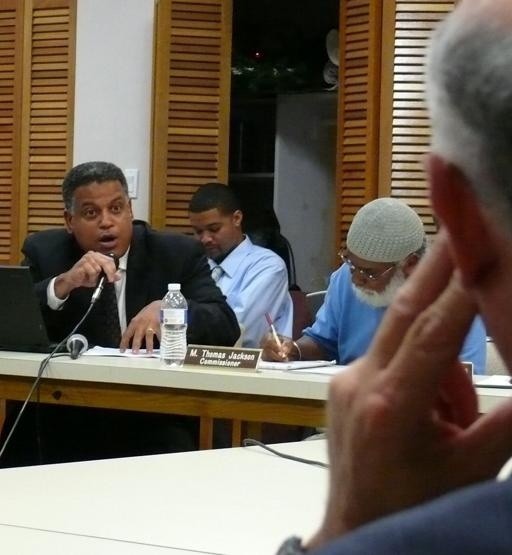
[346,197,424,264]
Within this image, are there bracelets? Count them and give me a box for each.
[292,341,302,361]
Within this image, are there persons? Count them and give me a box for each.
[259,196,487,374]
[19,160,242,356]
[274,1,512,555]
[188,182,295,349]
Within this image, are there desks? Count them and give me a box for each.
[0,351,512,452]
[0,438,330,554]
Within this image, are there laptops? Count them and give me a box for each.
[0,265,95,353]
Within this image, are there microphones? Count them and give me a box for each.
[67,334,88,359]
[91,252,119,300]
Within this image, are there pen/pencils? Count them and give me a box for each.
[265,313,289,364]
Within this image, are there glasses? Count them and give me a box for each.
[337,245,395,280]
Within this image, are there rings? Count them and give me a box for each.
[144,327,154,333]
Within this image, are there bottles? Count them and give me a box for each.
[158,283,189,367]
